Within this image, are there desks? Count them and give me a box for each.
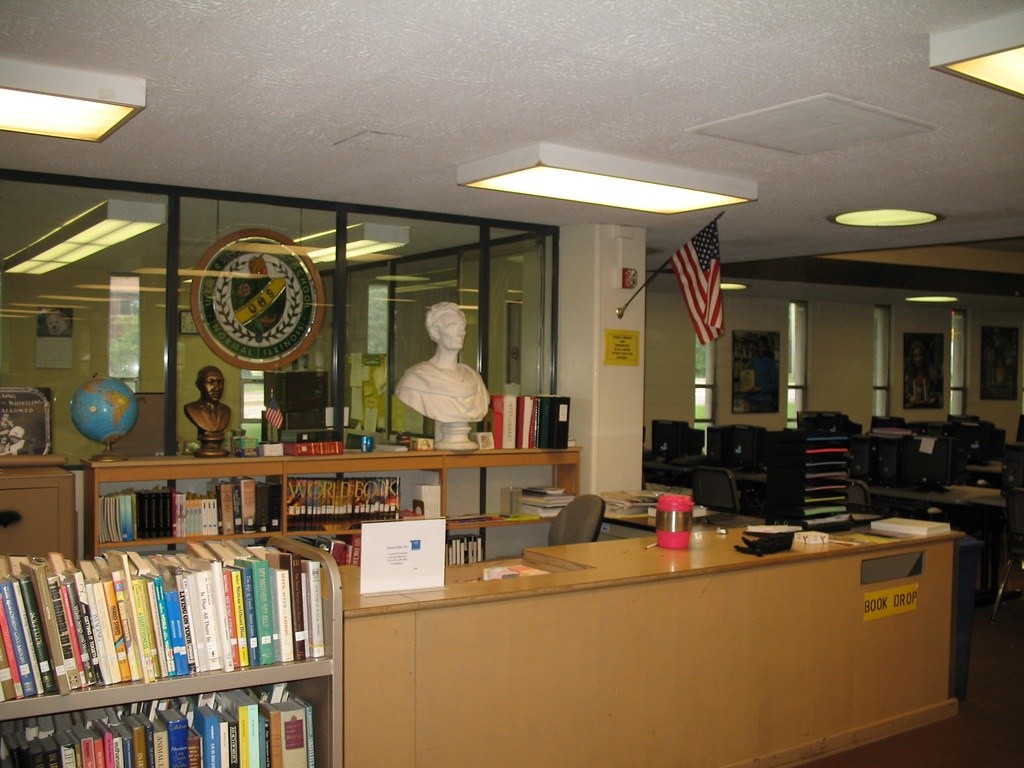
[643,455,1024,609]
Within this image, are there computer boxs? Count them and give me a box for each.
[850,435,878,483]
[879,436,904,489]
[707,423,732,468]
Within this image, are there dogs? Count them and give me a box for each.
[46,311,70,336]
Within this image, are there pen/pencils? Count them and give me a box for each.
[645,542,658,550]
[466,578,482,584]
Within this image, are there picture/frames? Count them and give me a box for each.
[732,330,780,414]
[979,326,1019,400]
[903,332,945,409]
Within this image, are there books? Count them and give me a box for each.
[98,476,282,542]
[287,476,441,532]
[0,537,325,701]
[871,517,951,536]
[297,535,361,567]
[732,330,781,412]
[748,525,803,533]
[602,489,707,516]
[516,486,577,516]
[0,681,315,768]
[444,534,483,565]
[914,378,926,403]
[953,419,979,426]
[484,394,575,449]
[873,427,911,434]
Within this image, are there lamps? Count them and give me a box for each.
[927,8,1024,100]
[295,223,411,263]
[1,199,165,275]
[0,57,146,143]
[455,142,758,214]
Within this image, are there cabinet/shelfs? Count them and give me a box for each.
[0,538,343,768]
[80,446,582,565]
[0,467,77,562]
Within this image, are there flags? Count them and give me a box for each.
[265,399,283,429]
[671,219,724,345]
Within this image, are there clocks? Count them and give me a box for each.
[179,310,199,334]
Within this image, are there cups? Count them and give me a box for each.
[656,494,695,548]
[500,487,522,516]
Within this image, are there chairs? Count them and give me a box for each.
[988,485,1024,623]
[691,465,742,514]
[485,494,605,562]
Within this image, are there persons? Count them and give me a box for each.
[741,336,777,412]
[0,413,33,456]
[394,302,489,421]
[905,342,939,406]
[184,366,232,431]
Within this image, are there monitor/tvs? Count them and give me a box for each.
[901,419,990,494]
[870,417,906,434]
[816,412,861,436]
[730,424,758,468]
[652,419,705,463]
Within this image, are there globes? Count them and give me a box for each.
[69,376,140,463]
